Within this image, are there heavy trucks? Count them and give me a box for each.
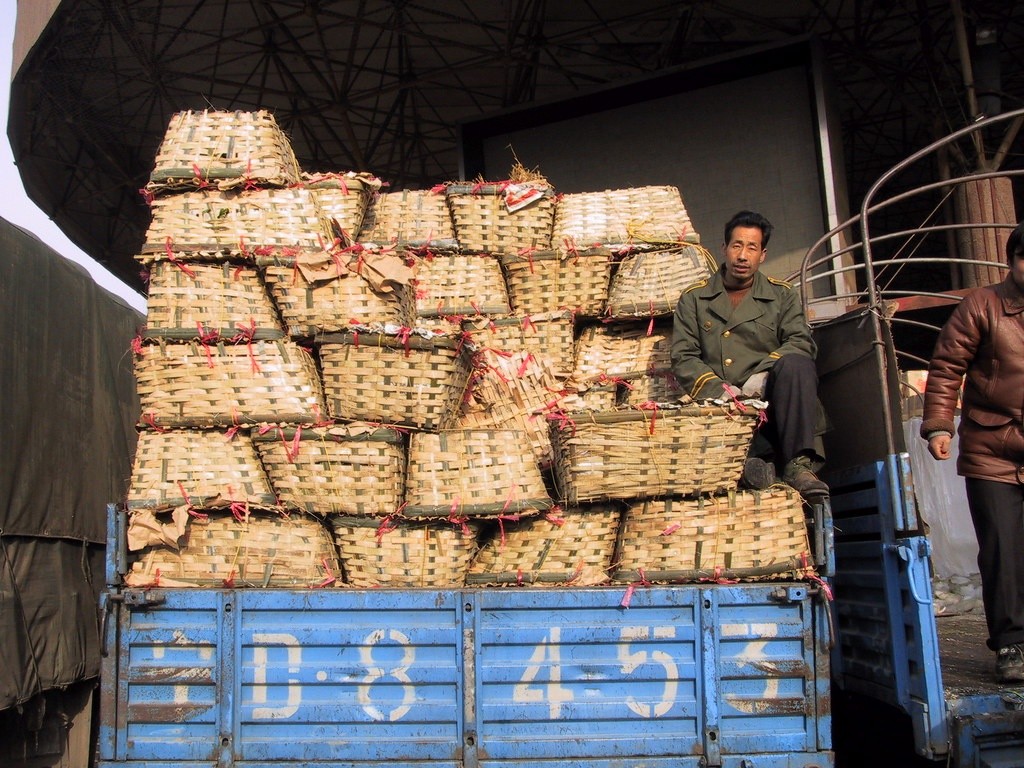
[0,215,148,768]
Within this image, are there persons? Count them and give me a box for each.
[920,222,1024,687]
[670,208,831,498]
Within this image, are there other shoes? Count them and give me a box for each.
[995,644,1024,680]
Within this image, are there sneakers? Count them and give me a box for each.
[741,458,776,489]
[783,456,829,498]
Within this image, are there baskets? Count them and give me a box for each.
[128,108,819,593]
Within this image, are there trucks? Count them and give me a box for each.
[781,106,1024,768]
[91,493,839,768]
